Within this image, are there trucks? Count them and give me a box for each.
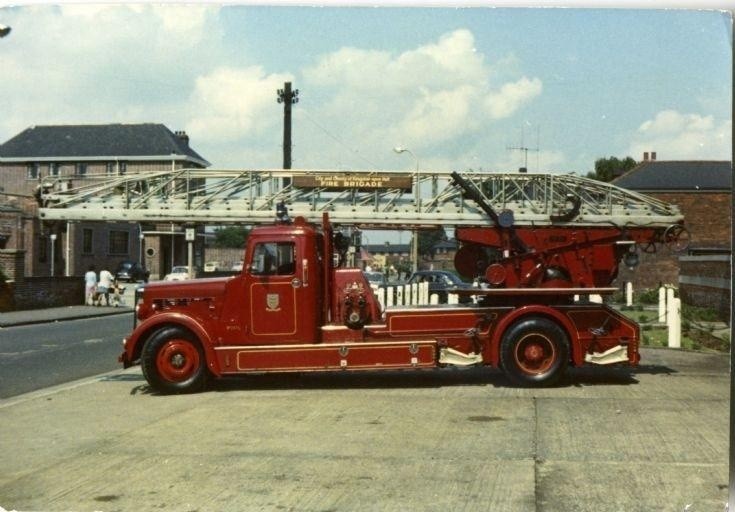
[37,172,691,395]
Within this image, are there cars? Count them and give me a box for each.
[164,266,189,281]
[204,262,219,274]
[231,261,244,274]
[364,272,384,289]
[407,270,473,288]
[116,260,150,283]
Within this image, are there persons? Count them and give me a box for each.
[95,266,116,307]
[83,264,98,306]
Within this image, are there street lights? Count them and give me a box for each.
[393,146,419,172]
[139,222,144,263]
[50,234,57,276]
[0,23,10,37]
